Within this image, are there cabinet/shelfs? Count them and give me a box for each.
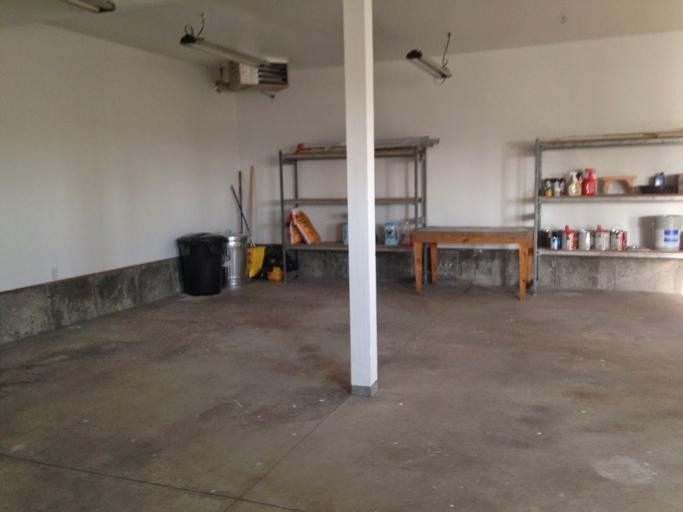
[278,145,427,285]
[531,131,683,297]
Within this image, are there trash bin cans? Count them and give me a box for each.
[175,233,229,296]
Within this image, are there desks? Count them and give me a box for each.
[411,225,533,302]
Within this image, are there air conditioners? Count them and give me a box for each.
[228,59,289,91]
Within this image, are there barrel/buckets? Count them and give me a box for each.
[653,173,666,193]
[176,231,226,295]
[222,233,244,286]
[649,215,683,251]
[398,221,411,245]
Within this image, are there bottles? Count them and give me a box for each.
[550,235,558,250]
[545,179,564,197]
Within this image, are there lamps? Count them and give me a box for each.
[405,30,452,80]
[179,12,271,69]
[62,0,116,14]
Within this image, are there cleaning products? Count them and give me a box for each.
[568,168,598,196]
